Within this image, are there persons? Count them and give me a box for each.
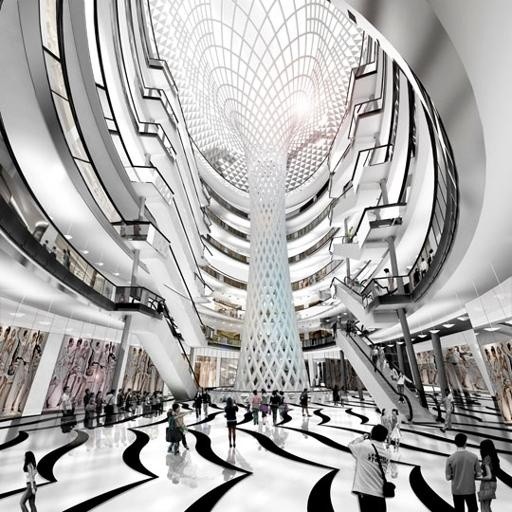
[164,451,182,480]
[427,249,435,267]
[0,325,165,455]
[383,266,395,295]
[377,345,388,372]
[419,257,428,279]
[329,317,362,341]
[394,370,415,404]
[345,426,395,512]
[436,387,455,433]
[412,268,421,286]
[220,449,238,480]
[328,383,341,407]
[20,450,40,511]
[444,431,479,510]
[301,417,311,438]
[474,436,501,512]
[63,248,72,272]
[369,343,380,372]
[165,384,287,455]
[388,451,400,478]
[299,388,312,417]
[338,385,349,407]
[172,449,189,484]
[43,239,49,252]
[51,245,57,260]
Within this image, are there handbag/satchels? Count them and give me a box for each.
[477,489,496,502]
[383,482,395,497]
[104,405,113,412]
[61,415,76,425]
